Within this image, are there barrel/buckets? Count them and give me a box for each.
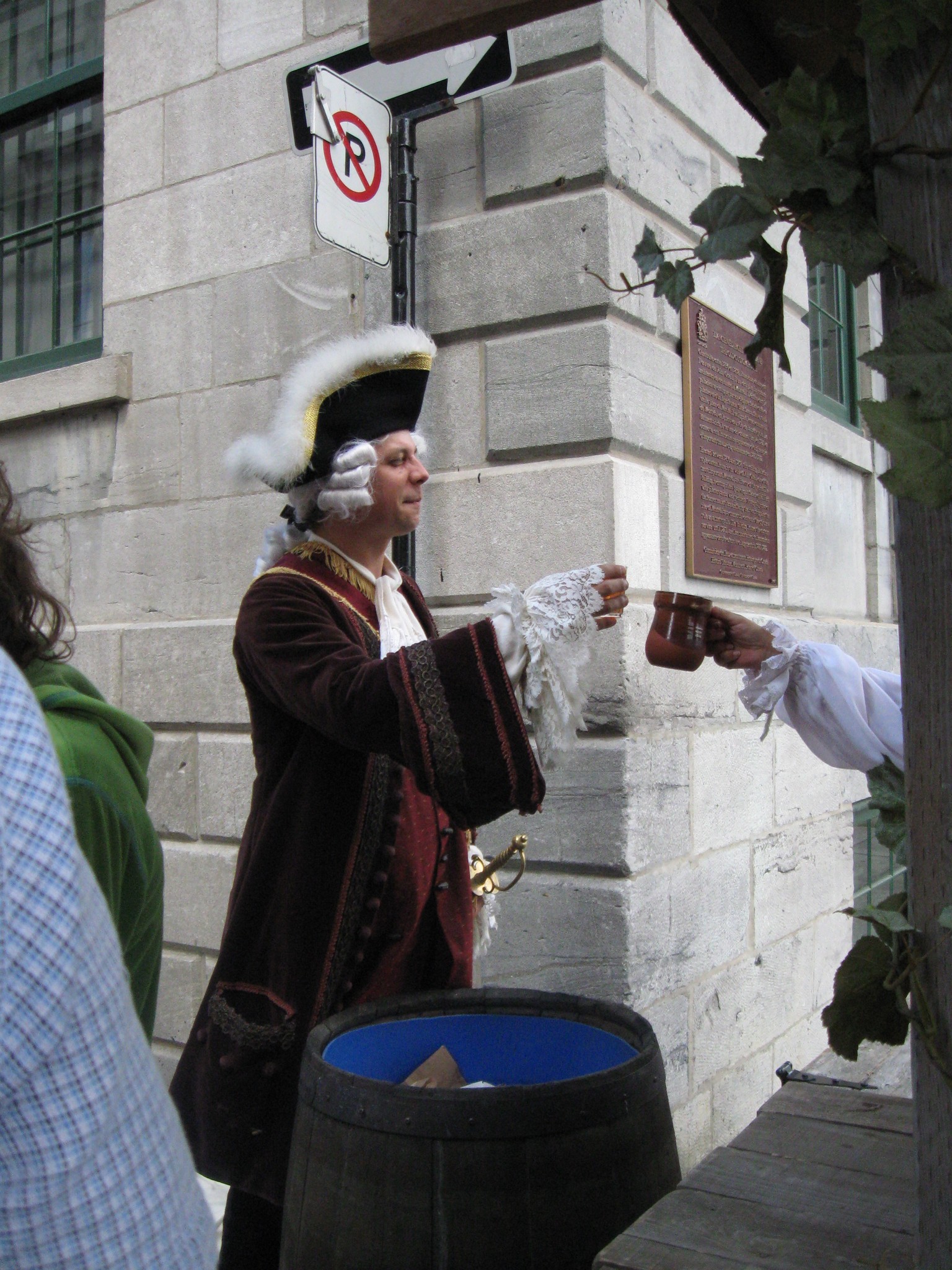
[279,988,683,1270]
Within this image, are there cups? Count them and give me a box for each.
[645,591,730,671]
[592,566,627,619]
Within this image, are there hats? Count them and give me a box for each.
[221,325,438,494]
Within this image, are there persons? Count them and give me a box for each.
[710,606,905,777]
[0,461,220,1270]
[168,327,630,1270]
